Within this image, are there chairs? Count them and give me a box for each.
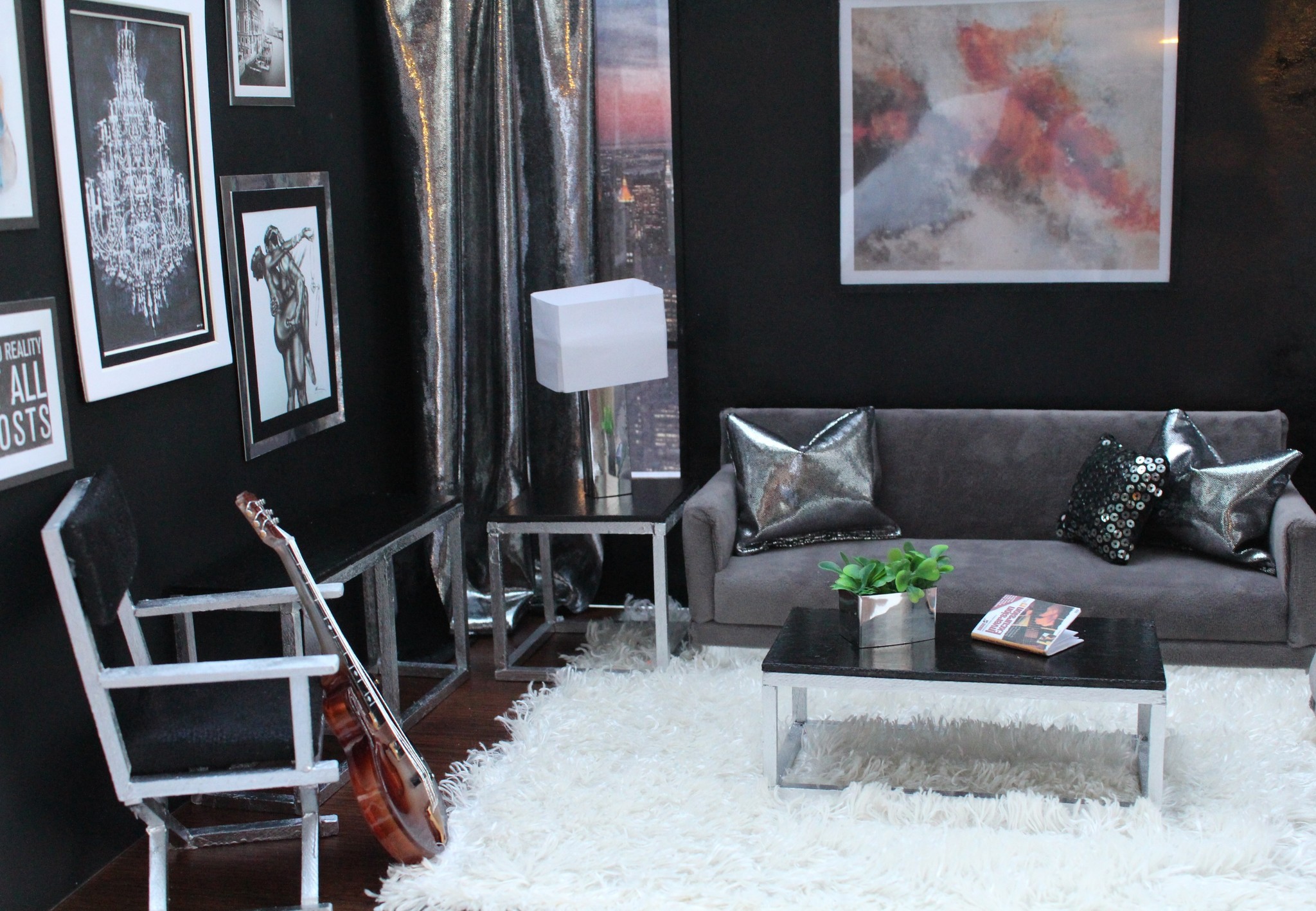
[42,471,353,909]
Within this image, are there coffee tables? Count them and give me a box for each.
[758,606,1169,803]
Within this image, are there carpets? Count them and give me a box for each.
[368,590,1315,911]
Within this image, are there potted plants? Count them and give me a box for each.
[815,539,958,648]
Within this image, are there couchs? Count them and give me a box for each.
[679,406,1316,671]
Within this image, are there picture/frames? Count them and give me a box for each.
[227,2,300,105]
[838,2,1179,287]
[217,165,360,466]
[1,1,49,237]
[42,1,246,396]
[1,295,75,495]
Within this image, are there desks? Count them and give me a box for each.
[485,475,702,686]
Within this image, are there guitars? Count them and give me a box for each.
[233,488,453,863]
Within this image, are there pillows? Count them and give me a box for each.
[1140,407,1305,578]
[725,408,910,554]
[1054,431,1172,563]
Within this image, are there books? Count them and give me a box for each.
[972,594,1085,656]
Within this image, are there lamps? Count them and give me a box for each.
[533,276,670,498]
